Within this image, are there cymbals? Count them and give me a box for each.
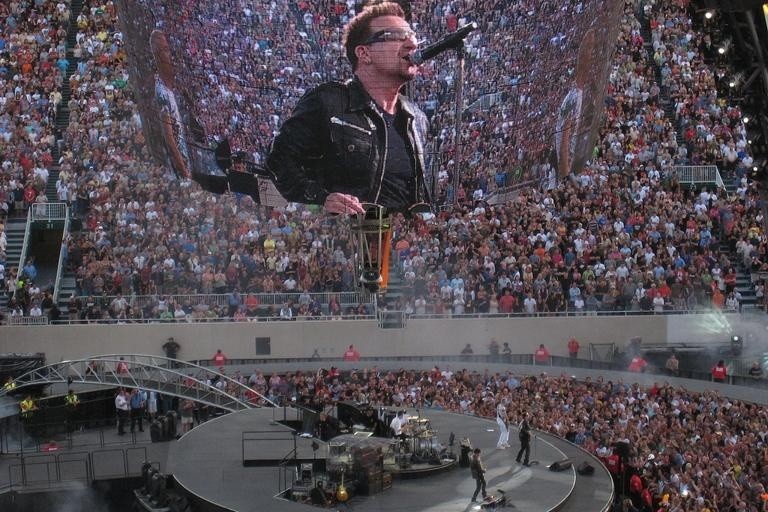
[407,416,429,422]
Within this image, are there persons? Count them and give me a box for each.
[567,335,580,366]
[128,386,146,433]
[63,388,81,431]
[458,344,474,362]
[178,362,768,510]
[711,359,727,382]
[161,336,181,368]
[211,348,229,366]
[116,357,129,375]
[488,340,500,362]
[343,344,361,361]
[534,344,550,364]
[113,387,130,436]
[19,392,40,420]
[1,1,767,330]
[501,342,512,361]
[747,360,764,380]
[666,351,680,375]
[4,375,17,392]
[626,352,652,373]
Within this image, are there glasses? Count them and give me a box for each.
[363,28,416,45]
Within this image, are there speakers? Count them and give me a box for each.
[549,460,571,472]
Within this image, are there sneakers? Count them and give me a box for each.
[504,445,510,447]
[130,430,135,432]
[472,499,477,503]
[140,430,144,431]
[523,464,531,467]
[119,433,125,436]
[496,446,504,449]
[515,459,521,463]
[483,495,487,497]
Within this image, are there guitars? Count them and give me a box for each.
[337,472,348,501]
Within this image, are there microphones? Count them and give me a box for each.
[409,21,477,63]
